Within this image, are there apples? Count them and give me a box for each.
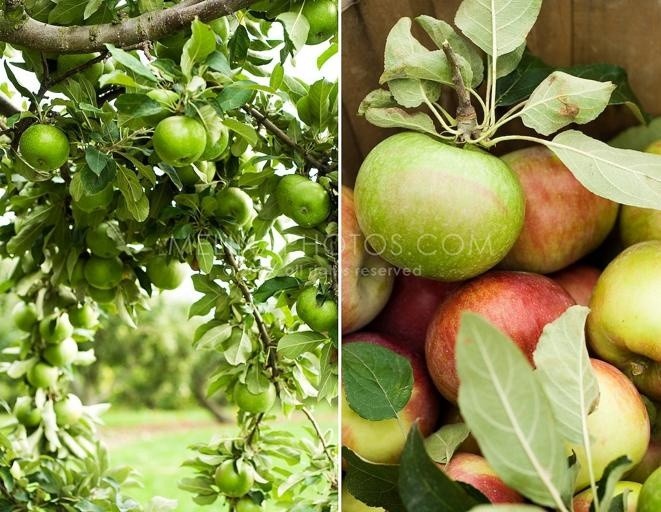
[16,1,338,511]
[341,132,661,512]
